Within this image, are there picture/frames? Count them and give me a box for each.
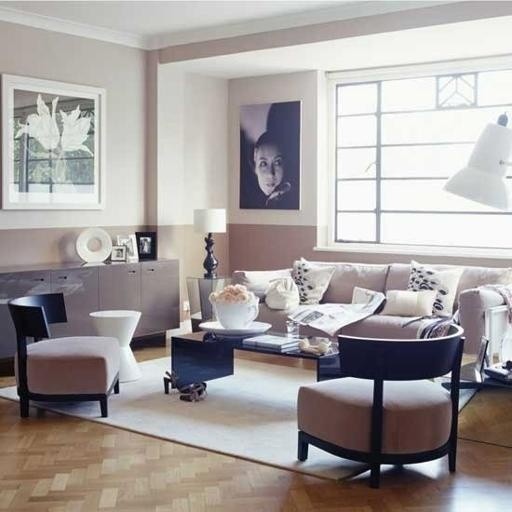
[238,99,301,210]
[111,245,127,262]
[117,234,139,262]
[0,73,106,211]
[135,231,158,259]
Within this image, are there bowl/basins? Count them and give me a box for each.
[216,297,259,329]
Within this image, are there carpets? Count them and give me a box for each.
[0,355,478,483]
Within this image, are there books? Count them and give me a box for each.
[242,335,302,353]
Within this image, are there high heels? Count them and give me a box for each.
[162,372,191,394]
[179,382,207,402]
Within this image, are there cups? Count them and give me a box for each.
[286,320,299,339]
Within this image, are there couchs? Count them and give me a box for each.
[230,263,512,382]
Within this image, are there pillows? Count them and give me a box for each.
[379,291,436,316]
[409,260,464,316]
[292,259,336,305]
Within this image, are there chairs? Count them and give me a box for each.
[9,293,122,418]
[298,319,467,484]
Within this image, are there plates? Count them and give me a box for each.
[198,321,272,335]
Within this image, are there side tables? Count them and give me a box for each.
[88,310,149,381]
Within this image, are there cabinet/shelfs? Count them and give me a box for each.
[0,261,99,357]
[99,260,180,337]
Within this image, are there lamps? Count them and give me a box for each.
[196,208,226,279]
[442,112,511,210]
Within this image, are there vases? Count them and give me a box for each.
[198,298,274,333]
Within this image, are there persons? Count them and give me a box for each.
[240,130,299,210]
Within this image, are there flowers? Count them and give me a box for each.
[206,284,253,301]
